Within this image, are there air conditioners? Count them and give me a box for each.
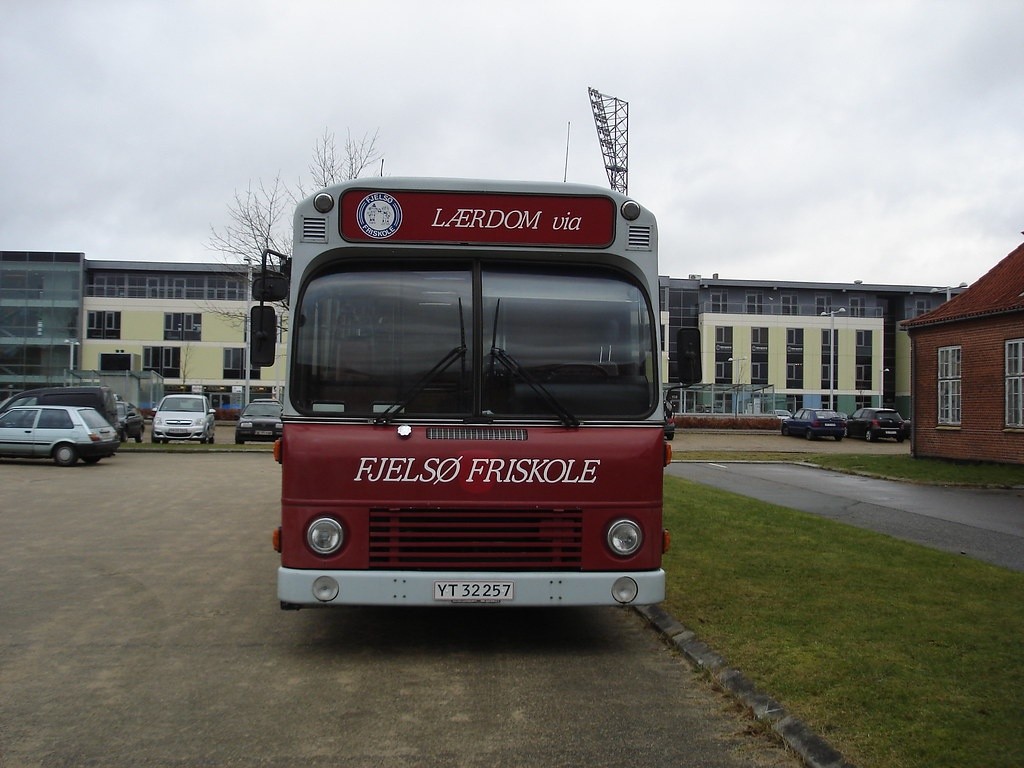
[689,274,701,279]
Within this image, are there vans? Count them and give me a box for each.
[0,384,118,433]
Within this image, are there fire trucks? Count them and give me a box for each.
[247,175,706,608]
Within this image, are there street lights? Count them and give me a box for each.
[241,254,258,413]
[821,307,846,409]
[64,339,81,386]
[728,356,748,419]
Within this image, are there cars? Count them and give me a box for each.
[116,400,145,443]
[234,398,284,445]
[0,405,120,467]
[767,410,793,421]
[664,399,675,440]
[781,408,846,441]
[844,407,906,442]
[151,392,217,445]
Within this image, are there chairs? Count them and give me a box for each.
[167,400,178,409]
[193,401,202,408]
[592,321,620,375]
[24,416,35,427]
